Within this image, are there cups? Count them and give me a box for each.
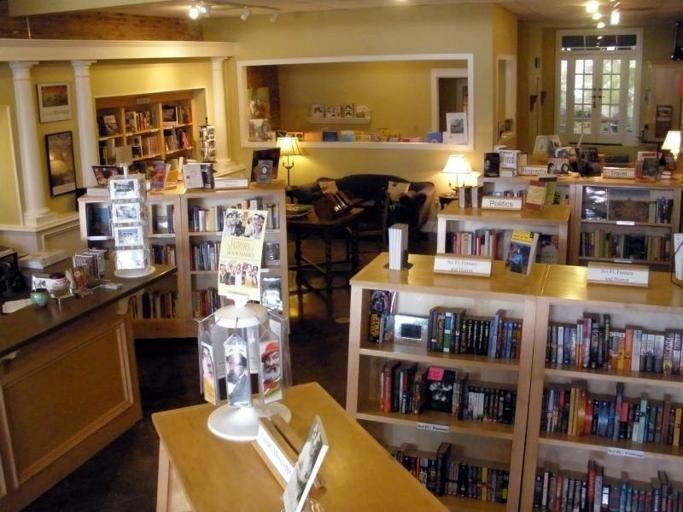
[674,233,683,282]
[31,288,50,309]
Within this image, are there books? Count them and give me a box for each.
[182,165,203,189]
[150,164,170,190]
[87,199,281,320]
[386,222,409,271]
[187,162,214,189]
[98,103,190,178]
[447,135,674,264]
[386,442,510,503]
[379,361,514,426]
[366,289,523,359]
[544,312,683,374]
[247,100,467,144]
[534,459,683,512]
[504,228,539,275]
[540,378,682,447]
[281,414,328,511]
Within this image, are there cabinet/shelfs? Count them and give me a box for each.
[78,186,289,340]
[91,87,213,185]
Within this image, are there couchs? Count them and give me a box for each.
[305,173,436,239]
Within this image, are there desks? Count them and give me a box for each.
[286,198,365,325]
[150,381,448,512]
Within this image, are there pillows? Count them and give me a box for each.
[386,181,411,204]
[317,179,339,194]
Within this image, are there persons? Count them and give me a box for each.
[199,345,214,380]
[227,353,247,402]
[262,343,283,392]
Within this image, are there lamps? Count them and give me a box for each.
[274,133,306,193]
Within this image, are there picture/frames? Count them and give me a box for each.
[35,81,73,125]
[43,129,79,199]
